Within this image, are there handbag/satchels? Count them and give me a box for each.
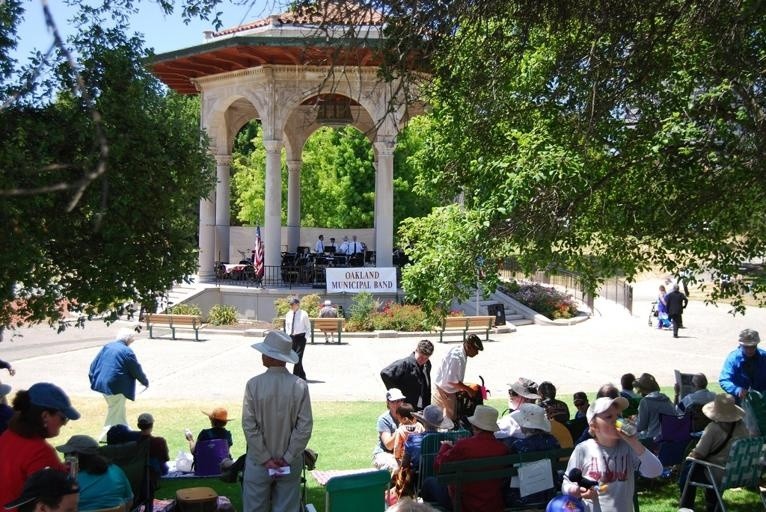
[684,439,698,465]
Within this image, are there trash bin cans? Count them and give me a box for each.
[487,303,507,326]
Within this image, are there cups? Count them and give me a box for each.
[64,457,79,482]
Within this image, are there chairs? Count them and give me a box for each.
[216,245,376,288]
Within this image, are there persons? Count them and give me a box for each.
[315,234,364,258]
[432,334,485,419]
[220,451,248,489]
[294,247,317,285]
[0,357,169,511]
[666,283,689,338]
[719,328,766,407]
[284,296,313,380]
[242,329,314,511]
[184,407,235,472]
[319,299,338,343]
[380,339,433,414]
[371,372,766,512]
[656,284,671,329]
[88,328,149,443]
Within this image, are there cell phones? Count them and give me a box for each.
[269,466,291,477]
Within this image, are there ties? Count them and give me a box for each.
[354,243,356,253]
[292,312,295,333]
[321,242,323,251]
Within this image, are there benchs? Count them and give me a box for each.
[433,316,497,343]
[280,318,345,345]
[143,312,203,340]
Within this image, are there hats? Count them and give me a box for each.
[287,299,299,304]
[0,383,234,509]
[250,331,298,364]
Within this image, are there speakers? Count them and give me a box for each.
[488,304,506,326]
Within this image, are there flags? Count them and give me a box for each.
[253,227,263,279]
